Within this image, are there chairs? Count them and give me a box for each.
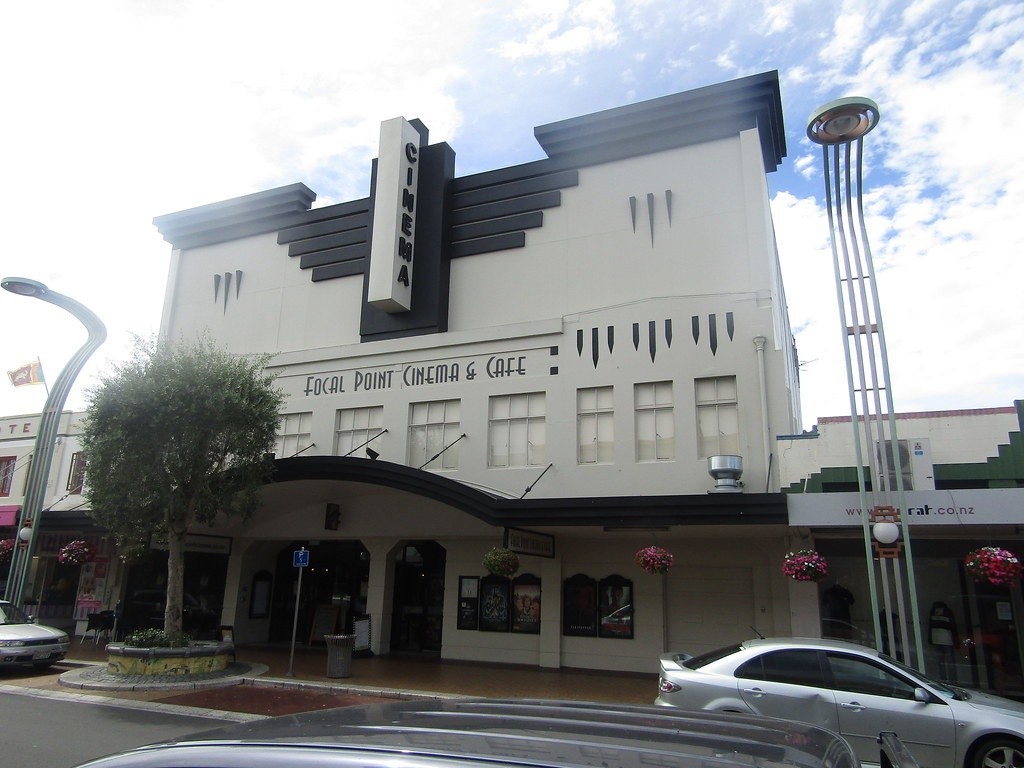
[79,610,115,645]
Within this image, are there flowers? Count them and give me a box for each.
[782,548,831,583]
[59,539,88,567]
[633,544,673,576]
[482,546,520,577]
[963,547,1023,587]
[0,539,14,563]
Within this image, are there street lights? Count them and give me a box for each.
[804,96,928,687]
[0,275,108,624]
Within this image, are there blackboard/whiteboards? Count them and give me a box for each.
[310,610,337,640]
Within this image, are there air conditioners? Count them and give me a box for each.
[874,437,936,492]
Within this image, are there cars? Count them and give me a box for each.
[0,599,71,673]
[72,696,920,767]
[653,638,1023,768]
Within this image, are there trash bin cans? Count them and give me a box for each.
[323,633,358,678]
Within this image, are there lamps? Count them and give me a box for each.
[19,524,31,541]
[873,515,898,544]
